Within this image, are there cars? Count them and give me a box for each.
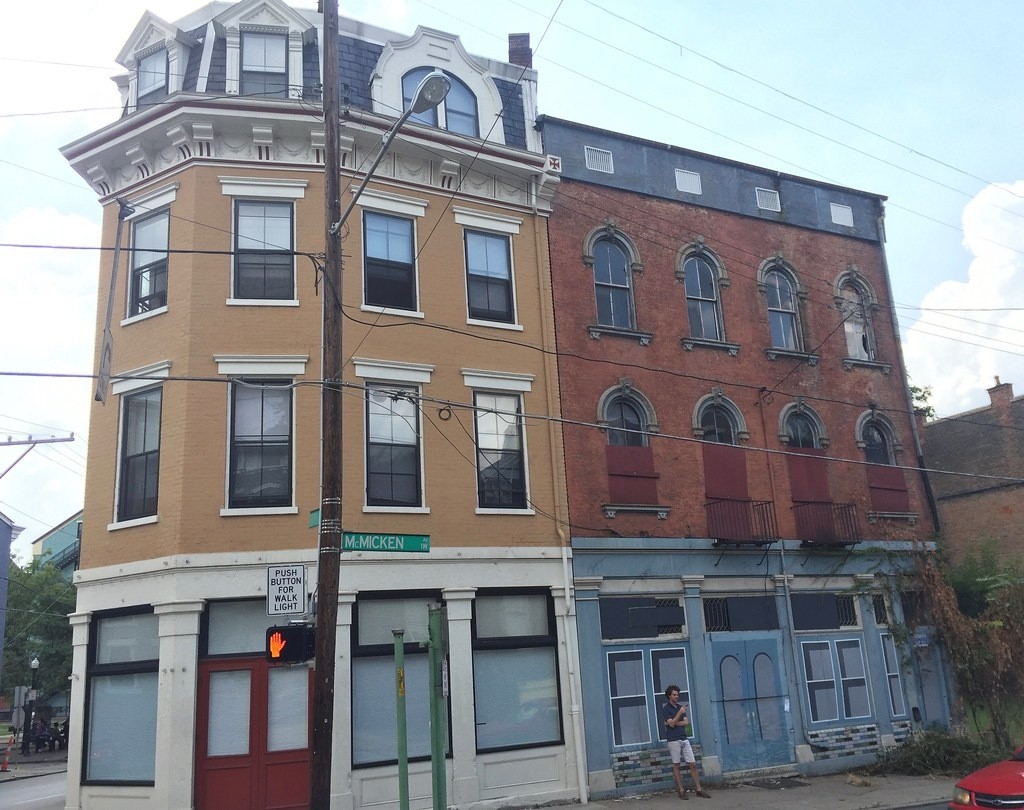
[947,745,1024,810]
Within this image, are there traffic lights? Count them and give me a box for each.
[265,625,315,664]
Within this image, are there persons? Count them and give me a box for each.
[662,685,711,800]
[35,716,70,753]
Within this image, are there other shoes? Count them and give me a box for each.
[679,791,689,800]
[697,789,711,798]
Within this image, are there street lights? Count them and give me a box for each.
[22,656,43,757]
[303,68,454,810]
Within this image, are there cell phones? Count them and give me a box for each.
[684,704,688,707]
